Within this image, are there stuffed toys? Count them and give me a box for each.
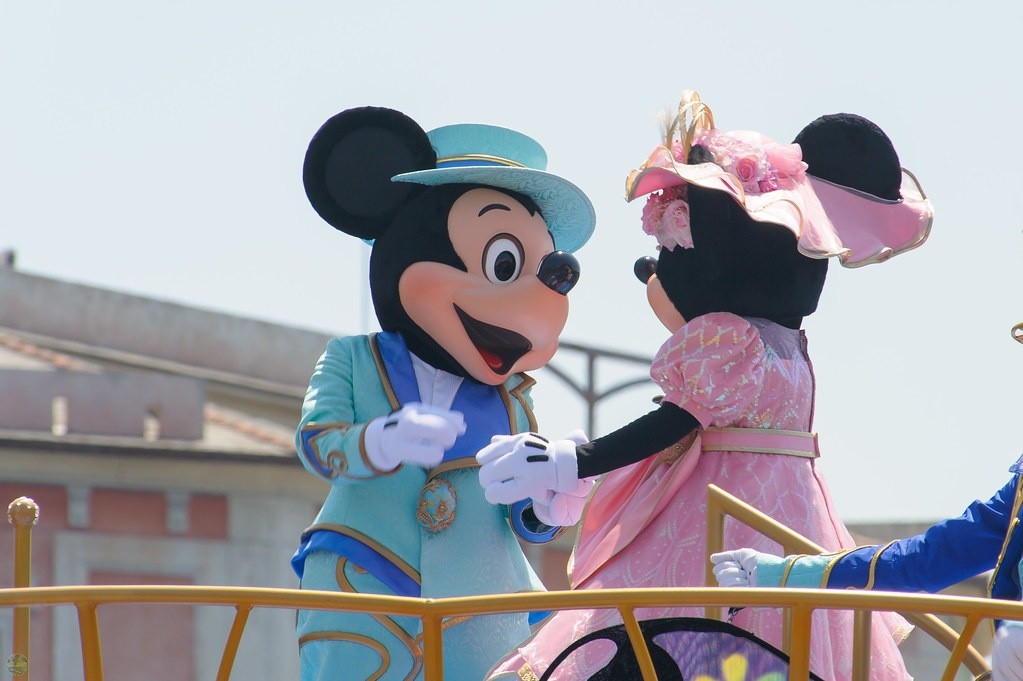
[709,320,1023,681]
[473,90,933,681]
[292,107,598,681]
